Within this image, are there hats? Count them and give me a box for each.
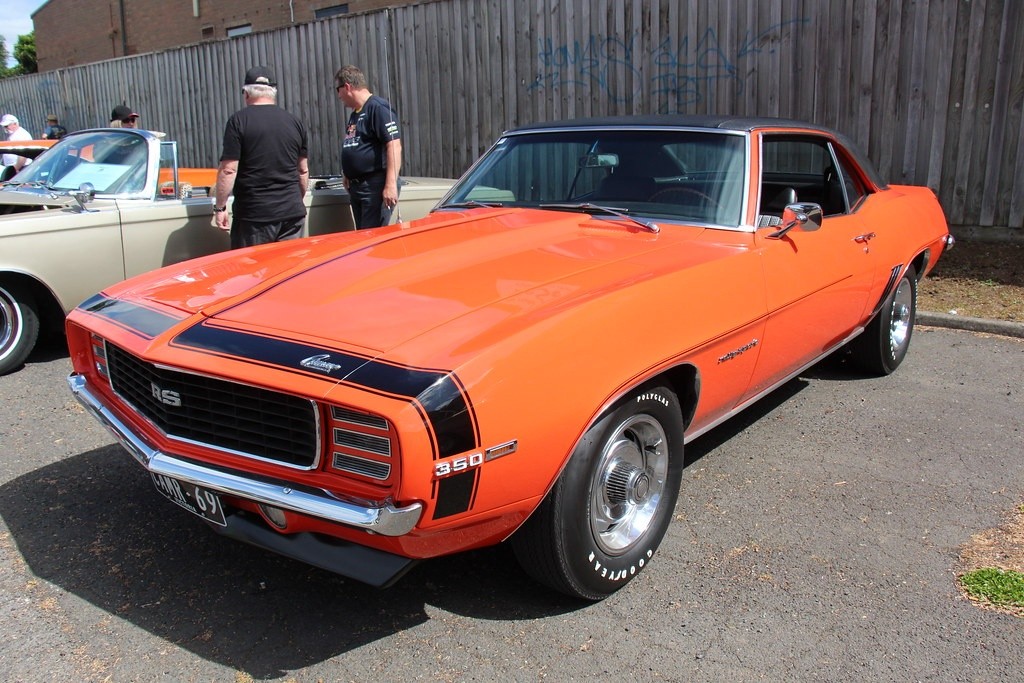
[241,66,275,93]
[45,114,57,122]
[0,113,18,127]
[112,105,140,123]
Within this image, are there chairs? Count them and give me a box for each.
[592,176,658,203]
[156,180,192,201]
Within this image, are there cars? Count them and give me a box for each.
[0,142,220,199]
[1,127,518,377]
[62,118,957,600]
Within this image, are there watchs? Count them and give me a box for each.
[213,205,226,212]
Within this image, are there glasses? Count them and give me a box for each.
[4,126,8,128]
[121,118,135,123]
[336,85,345,93]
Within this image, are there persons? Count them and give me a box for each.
[0,114,33,174]
[92,105,140,165]
[41,114,67,139]
[212,66,310,250]
[334,65,404,231]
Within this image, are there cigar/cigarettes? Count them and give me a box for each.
[387,205,391,210]
